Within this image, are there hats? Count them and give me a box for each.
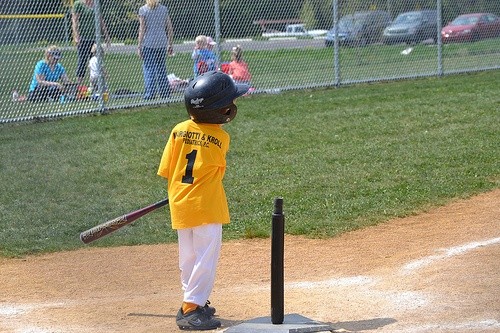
[49,51,64,59]
[207,36,217,45]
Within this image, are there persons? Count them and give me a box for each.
[70,0,111,85]
[89,45,109,100]
[192,35,251,89]
[28,46,71,103]
[157,71,250,330]
[137,0,175,100]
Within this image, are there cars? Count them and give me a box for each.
[441,12,500,44]
[325,10,390,47]
[382,10,437,46]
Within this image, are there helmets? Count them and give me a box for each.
[184,71,249,124]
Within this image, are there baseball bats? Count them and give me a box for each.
[79,198,168,244]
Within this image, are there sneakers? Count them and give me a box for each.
[203,300,215,316]
[175,306,221,330]
